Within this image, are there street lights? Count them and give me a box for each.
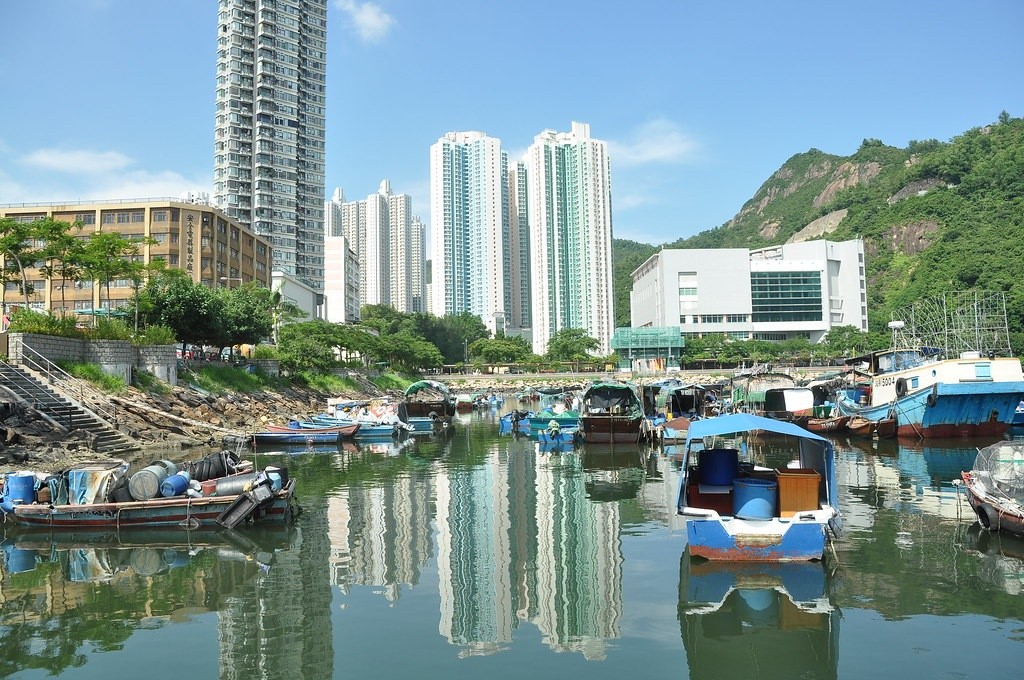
[220,276,243,287]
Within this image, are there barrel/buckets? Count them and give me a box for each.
[7,470,36,505]
[260,464,288,487]
[202,482,216,497]
[699,448,739,485]
[8,545,35,574]
[169,555,189,568]
[129,465,167,501]
[149,459,179,477]
[738,588,775,610]
[733,477,778,519]
[160,474,189,497]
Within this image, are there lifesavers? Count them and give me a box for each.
[977,502,998,531]
[887,409,898,425]
[927,393,936,407]
[977,529,1002,555]
[896,378,907,398]
[180,518,202,525]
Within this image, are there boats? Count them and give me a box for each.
[0,450,303,530]
[253,397,401,443]
[403,380,456,433]
[455,387,504,410]
[499,346,1024,442]
[675,413,845,563]
[960,470,1024,536]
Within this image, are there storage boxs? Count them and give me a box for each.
[774,467,821,517]
